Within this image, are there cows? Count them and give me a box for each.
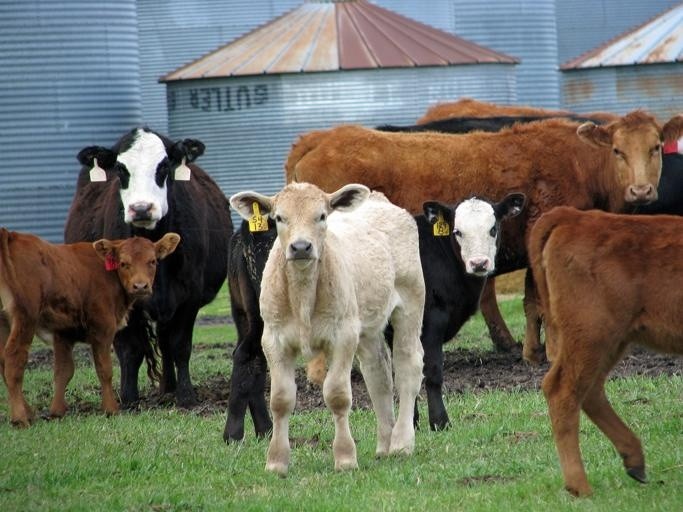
[0,228,181,429]
[63,126,234,415]
[527,204,683,499]
[228,182,428,480]
[282,96,683,366]
[222,190,527,443]
